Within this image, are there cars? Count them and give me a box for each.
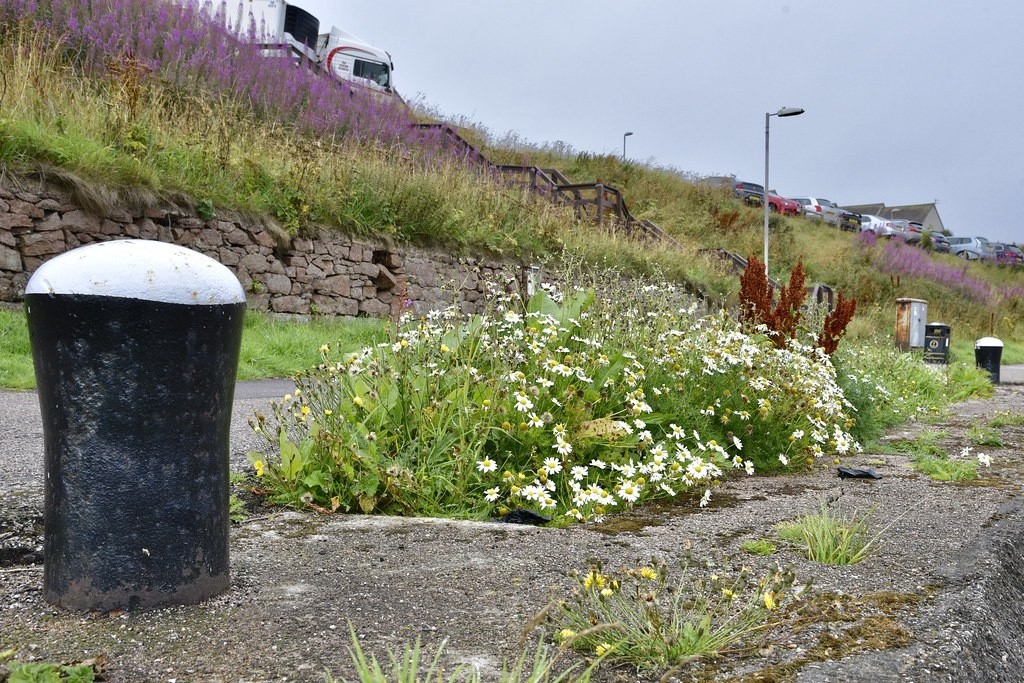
[699,175,764,208]
[786,197,1024,268]
[768,189,803,217]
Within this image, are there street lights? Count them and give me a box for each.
[623,132,633,164]
[890,208,902,221]
[763,105,806,296]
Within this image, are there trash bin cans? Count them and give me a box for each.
[923,322,952,365]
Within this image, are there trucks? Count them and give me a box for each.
[197,0,394,108]
